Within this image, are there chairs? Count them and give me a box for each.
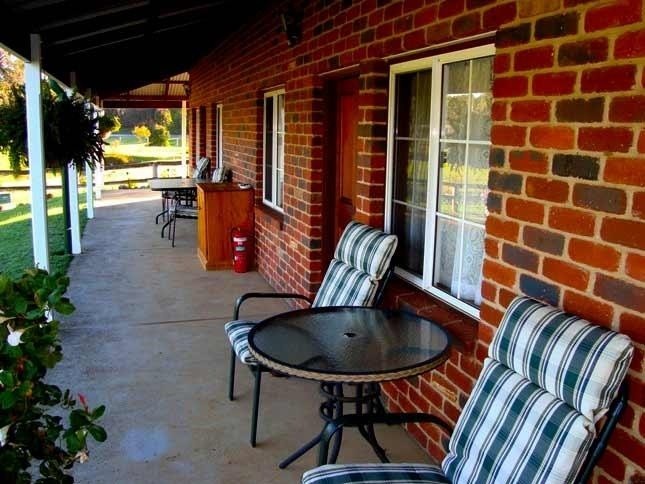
[223,212,403,449]
[162,156,210,221]
[301,293,637,484]
[167,166,230,243]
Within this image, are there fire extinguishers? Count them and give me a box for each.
[230,223,254,273]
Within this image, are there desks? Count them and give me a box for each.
[248,303,454,474]
[149,176,213,239]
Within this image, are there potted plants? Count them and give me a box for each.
[0,78,108,175]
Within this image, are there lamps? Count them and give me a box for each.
[277,0,303,48]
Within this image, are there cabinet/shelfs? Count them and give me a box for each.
[192,179,256,271]
